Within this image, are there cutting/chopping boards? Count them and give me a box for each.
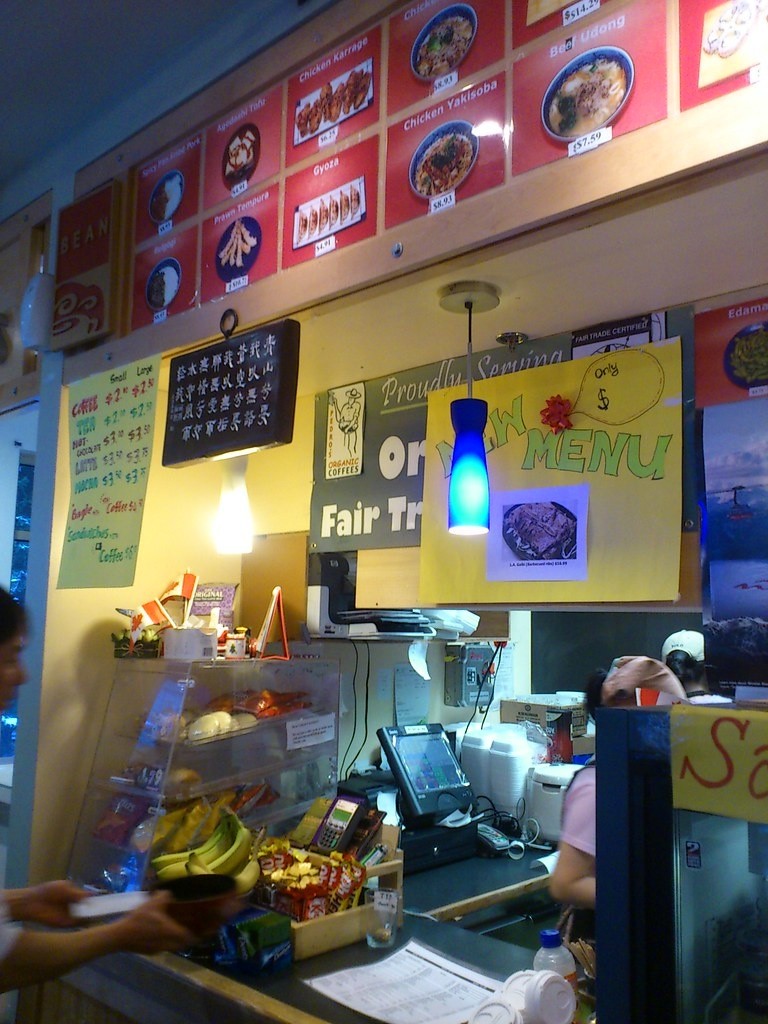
[697,0,768,89]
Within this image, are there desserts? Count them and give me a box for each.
[184,710,259,743]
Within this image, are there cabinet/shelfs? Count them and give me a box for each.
[56,653,340,897]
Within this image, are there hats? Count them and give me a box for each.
[661,629,705,664]
[601,655,688,704]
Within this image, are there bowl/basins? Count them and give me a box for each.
[408,120,480,199]
[152,873,239,930]
[502,502,578,561]
[410,2,477,80]
[540,45,634,142]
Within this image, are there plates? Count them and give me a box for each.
[294,57,374,146]
[215,216,262,281]
[149,169,184,223]
[292,175,367,249]
[146,257,182,311]
[221,121,261,190]
[68,891,150,917]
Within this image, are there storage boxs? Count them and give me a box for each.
[164,629,217,658]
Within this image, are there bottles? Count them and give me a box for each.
[533,929,582,1024]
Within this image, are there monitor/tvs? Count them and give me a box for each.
[376,723,479,818]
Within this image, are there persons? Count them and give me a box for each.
[547,656,690,964]
[0,586,220,994]
[661,629,735,704]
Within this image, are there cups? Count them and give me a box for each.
[364,887,400,948]
[224,636,245,660]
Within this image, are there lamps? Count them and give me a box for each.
[215,453,254,554]
[437,282,500,535]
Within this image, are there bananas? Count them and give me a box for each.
[150,805,266,897]
